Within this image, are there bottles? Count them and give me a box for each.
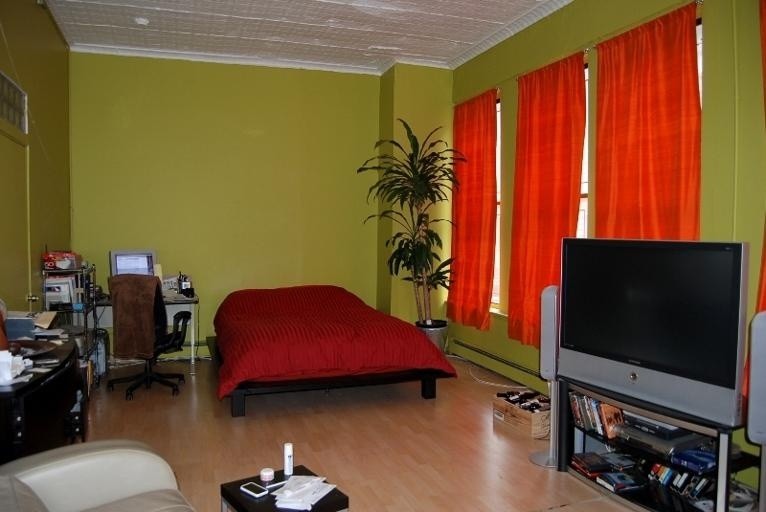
[283,442,294,476]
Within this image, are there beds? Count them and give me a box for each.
[205,285,457,417]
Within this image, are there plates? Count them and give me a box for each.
[10,340,56,358]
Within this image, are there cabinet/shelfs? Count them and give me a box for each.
[550,374,763,510]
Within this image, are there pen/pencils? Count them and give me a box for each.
[178,271,191,282]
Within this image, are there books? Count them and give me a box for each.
[44,273,91,310]
[270,475,337,512]
[567,391,713,502]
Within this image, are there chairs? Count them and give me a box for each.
[107,274,192,400]
[0,436,200,512]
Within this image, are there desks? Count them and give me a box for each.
[45,288,198,368]
[0,326,85,448]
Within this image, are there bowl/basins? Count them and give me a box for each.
[56,260,72,270]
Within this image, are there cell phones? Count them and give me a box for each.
[240,482,269,498]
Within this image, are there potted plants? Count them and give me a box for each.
[355,116,468,356]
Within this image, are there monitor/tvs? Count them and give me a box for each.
[557,238,749,427]
[109,250,158,276]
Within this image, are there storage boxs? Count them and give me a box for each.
[492,386,551,439]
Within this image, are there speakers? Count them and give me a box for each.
[539,286,560,382]
[745,310,766,447]
